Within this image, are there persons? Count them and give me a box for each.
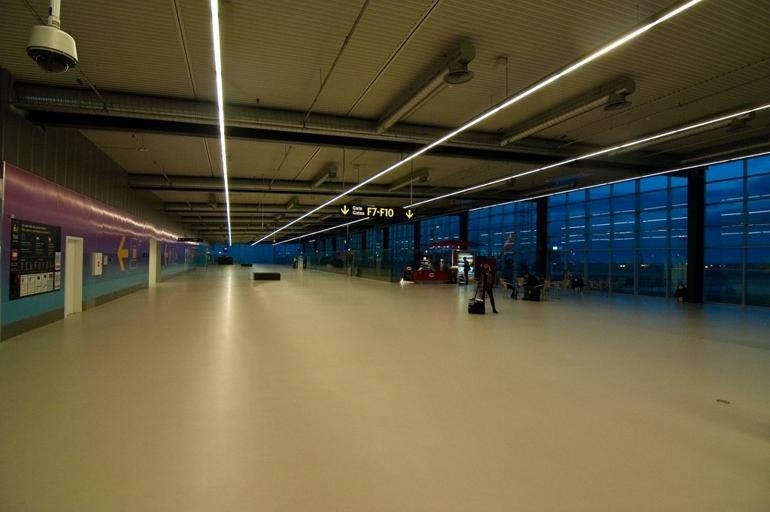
[293,255,307,269]
[460,257,471,285]
[472,262,498,314]
[522,271,541,298]
[568,273,585,297]
[503,276,519,300]
[468,264,484,301]
